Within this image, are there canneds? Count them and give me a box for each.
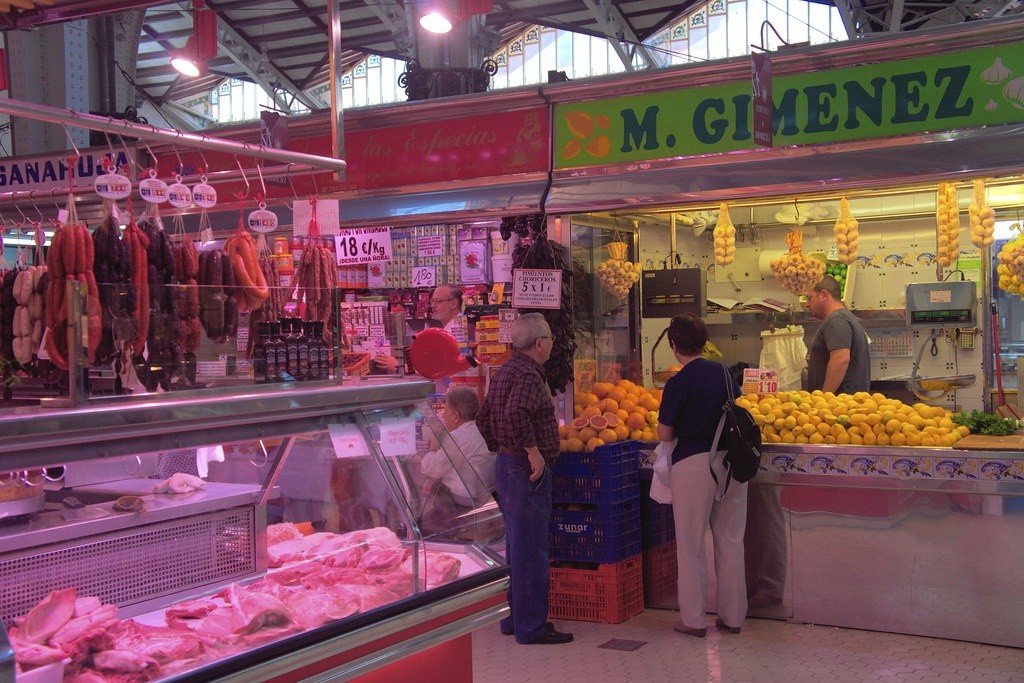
[273,235,368,302]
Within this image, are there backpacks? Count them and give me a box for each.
[709,364,762,501]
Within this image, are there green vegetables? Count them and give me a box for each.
[950,409,1024,436]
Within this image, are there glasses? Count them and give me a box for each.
[430,298,460,304]
[540,333,556,341]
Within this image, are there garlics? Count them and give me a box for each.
[997,240,1024,294]
[596,259,642,299]
[968,203,995,249]
[770,255,827,292]
[937,186,960,267]
[713,226,735,266]
[833,221,859,263]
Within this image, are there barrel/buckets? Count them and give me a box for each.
[490,230,519,283]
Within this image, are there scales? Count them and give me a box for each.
[641,266,707,390]
[904,269,984,401]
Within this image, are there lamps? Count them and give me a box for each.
[417,0,458,34]
[169,36,206,78]
[258,4,269,62]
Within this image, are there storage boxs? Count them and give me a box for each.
[342,352,370,376]
[548,497,641,565]
[458,228,472,240]
[642,540,676,604]
[547,552,646,624]
[552,440,642,502]
[456,239,492,284]
[472,226,489,240]
[643,495,675,549]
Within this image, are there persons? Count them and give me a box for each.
[476,312,573,644]
[656,312,749,636]
[373,285,468,375]
[805,275,871,397]
[420,388,494,515]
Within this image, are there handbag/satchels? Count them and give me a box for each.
[649,438,679,503]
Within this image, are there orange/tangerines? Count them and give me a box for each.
[824,263,847,299]
[735,390,970,446]
[559,380,664,452]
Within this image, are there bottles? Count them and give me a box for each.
[271,237,294,287]
[253,317,329,384]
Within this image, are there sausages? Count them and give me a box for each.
[0,215,281,392]
[283,244,338,325]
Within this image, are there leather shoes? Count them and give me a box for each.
[503,622,574,644]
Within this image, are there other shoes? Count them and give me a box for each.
[715,618,741,633]
[673,621,706,637]
